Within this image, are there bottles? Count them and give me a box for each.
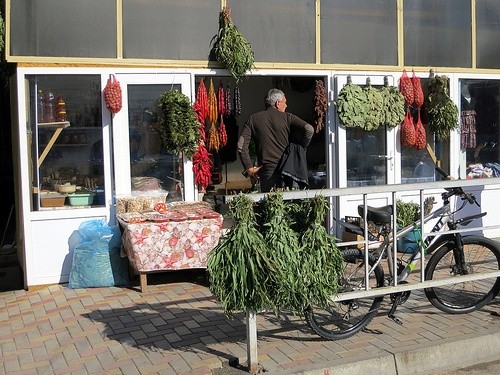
[37,89,47,123]
[45,92,56,122]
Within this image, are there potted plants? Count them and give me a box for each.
[396,200,423,254]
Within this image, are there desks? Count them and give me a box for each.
[117,207,224,295]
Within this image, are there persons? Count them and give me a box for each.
[238,89,314,193]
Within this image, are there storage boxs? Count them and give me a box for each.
[342,229,393,254]
[130,189,168,207]
[93,189,105,205]
[66,191,96,206]
[39,193,67,207]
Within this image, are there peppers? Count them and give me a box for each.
[190,102,214,192]
